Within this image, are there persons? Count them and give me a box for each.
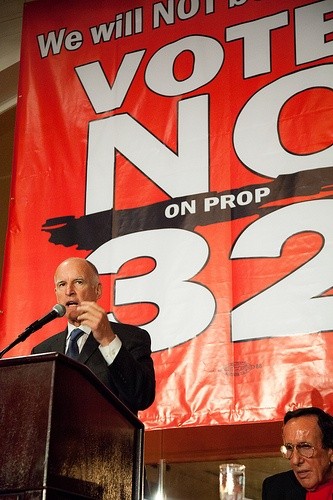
[30,259,155,420]
[261,408,333,500]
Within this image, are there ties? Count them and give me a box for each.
[63,327,84,360]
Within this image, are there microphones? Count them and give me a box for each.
[19,303,67,343]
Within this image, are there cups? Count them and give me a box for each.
[219,463,247,500]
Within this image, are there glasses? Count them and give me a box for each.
[280,440,324,460]
